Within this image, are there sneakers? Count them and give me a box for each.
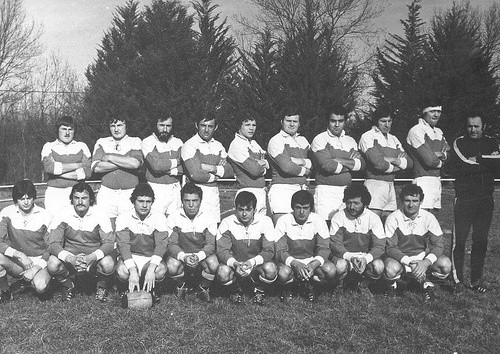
[59,282,75,303]
[422,289,443,306]
[469,283,490,295]
[305,289,317,304]
[385,285,399,301]
[280,288,294,303]
[358,287,375,302]
[229,289,245,306]
[0,288,14,304]
[146,290,161,302]
[119,287,129,300]
[252,285,267,305]
[331,283,345,301]
[196,285,214,303]
[451,283,467,298]
[171,283,187,301]
[94,287,108,305]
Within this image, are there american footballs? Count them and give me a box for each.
[121,290,157,308]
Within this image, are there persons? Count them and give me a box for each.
[0,180,51,297]
[138,110,185,237]
[449,110,499,294]
[275,188,337,304]
[181,109,235,249]
[328,181,387,297]
[214,190,280,305]
[228,110,269,226]
[267,110,314,233]
[402,97,452,224]
[164,182,218,303]
[47,181,117,304]
[308,105,364,244]
[355,105,416,231]
[90,114,145,238]
[114,182,168,302]
[381,184,453,302]
[39,115,92,216]
